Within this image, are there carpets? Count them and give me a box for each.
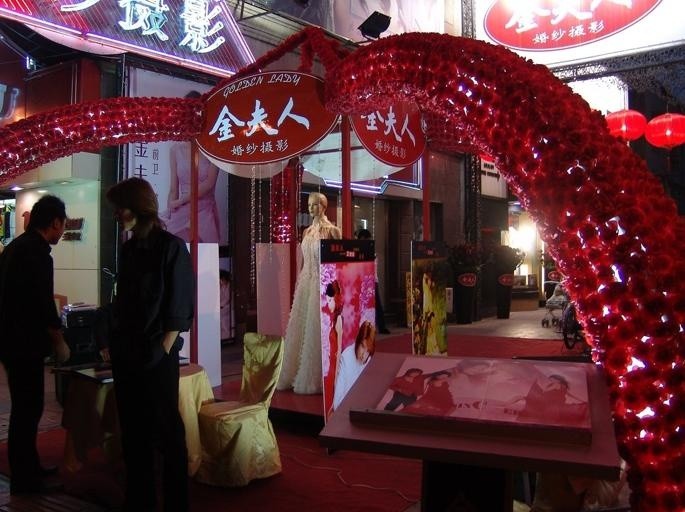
[0,333,586,512]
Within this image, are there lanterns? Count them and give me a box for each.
[642,111,685,150]
[604,107,649,146]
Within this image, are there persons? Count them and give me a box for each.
[274,190,343,394]
[379,354,590,432]
[545,284,566,328]
[320,278,343,420]
[1,193,78,501]
[333,320,376,414]
[92,175,197,509]
[418,270,436,354]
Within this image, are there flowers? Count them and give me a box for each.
[452,242,524,270]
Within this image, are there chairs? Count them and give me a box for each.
[199,333,286,487]
[55,310,102,409]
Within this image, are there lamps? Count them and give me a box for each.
[355,12,392,44]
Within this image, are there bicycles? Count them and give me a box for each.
[560,300,578,350]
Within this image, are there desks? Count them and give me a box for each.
[63,363,204,477]
[320,353,621,512]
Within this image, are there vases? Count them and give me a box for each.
[453,264,514,324]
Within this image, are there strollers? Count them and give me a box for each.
[540,280,566,328]
